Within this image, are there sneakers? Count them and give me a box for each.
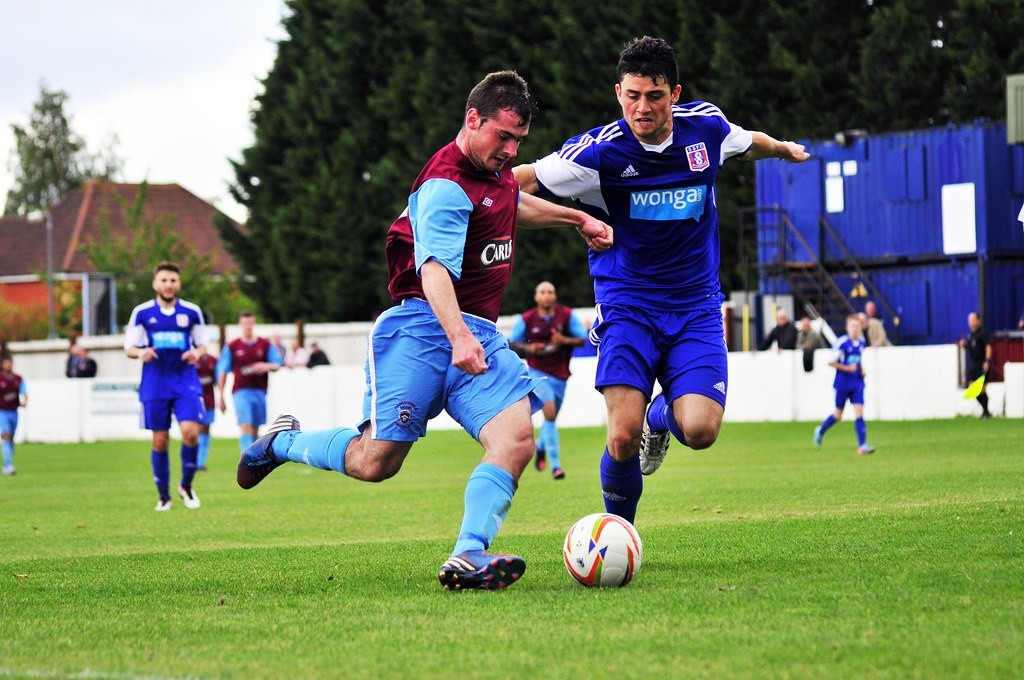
[856,445,875,455]
[815,425,823,447]
[439,552,525,592]
[155,498,173,511]
[536,446,546,472]
[640,389,671,476]
[178,485,201,507]
[237,415,299,489]
[553,468,563,479]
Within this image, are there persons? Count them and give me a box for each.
[124,261,210,510]
[66,344,96,378]
[0,357,29,476]
[755,301,895,353]
[197,310,329,471]
[508,281,588,479]
[511,36,811,526]
[957,311,995,418]
[238,71,613,589]
[815,314,878,455]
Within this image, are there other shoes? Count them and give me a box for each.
[980,413,995,419]
[2,465,16,476]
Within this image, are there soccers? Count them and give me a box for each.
[563,514,642,588]
